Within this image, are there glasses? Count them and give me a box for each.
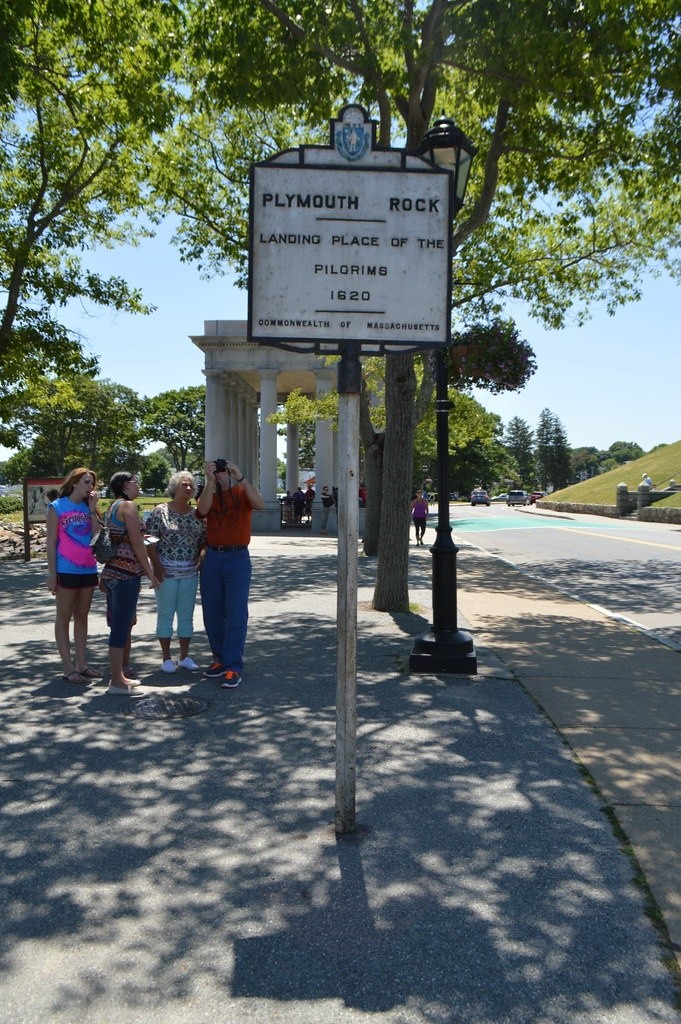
[128,480,139,487]
[417,492,423,493]
[325,489,328,491]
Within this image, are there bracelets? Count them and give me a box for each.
[238,476,244,482]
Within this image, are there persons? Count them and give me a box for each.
[412,491,429,545]
[423,491,435,506]
[320,486,333,533]
[197,460,265,687]
[284,485,315,522]
[642,473,653,488]
[145,472,207,672]
[46,468,104,683]
[99,472,160,695]
[360,483,367,506]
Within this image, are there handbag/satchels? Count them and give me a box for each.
[323,496,334,507]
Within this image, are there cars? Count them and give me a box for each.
[492,489,548,507]
[471,490,491,506]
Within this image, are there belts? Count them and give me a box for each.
[208,543,248,552]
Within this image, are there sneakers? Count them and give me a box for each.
[177,657,199,670]
[222,670,243,687]
[162,659,177,673]
[108,684,143,695]
[203,662,228,677]
[109,678,141,688]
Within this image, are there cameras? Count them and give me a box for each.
[212,459,228,473]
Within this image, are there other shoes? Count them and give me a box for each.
[416,544,419,546]
[419,538,423,545]
[110,667,136,677]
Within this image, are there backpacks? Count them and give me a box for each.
[89,512,129,563]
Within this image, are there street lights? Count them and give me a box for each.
[409,114,482,676]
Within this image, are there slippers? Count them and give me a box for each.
[62,670,87,683]
[76,666,103,678]
